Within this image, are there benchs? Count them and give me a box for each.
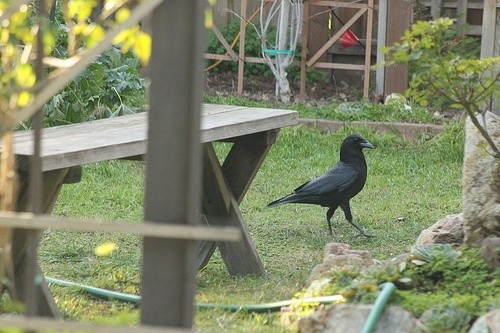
[3,103,300,331]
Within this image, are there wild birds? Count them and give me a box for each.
[266,134,376,239]
